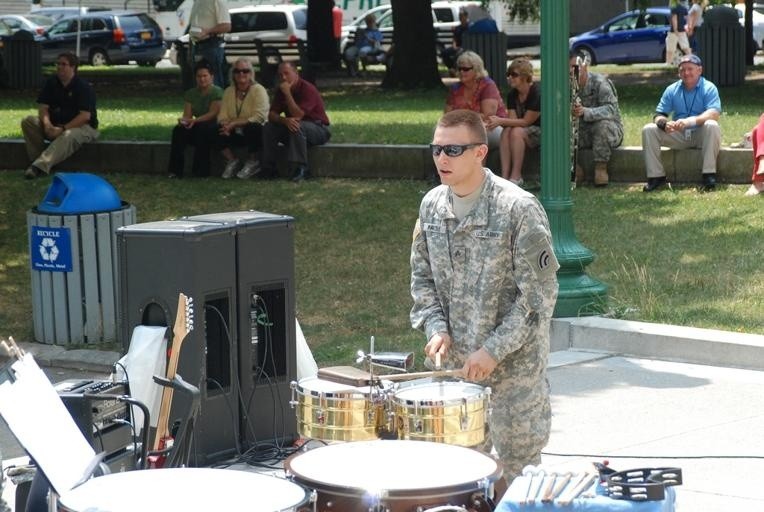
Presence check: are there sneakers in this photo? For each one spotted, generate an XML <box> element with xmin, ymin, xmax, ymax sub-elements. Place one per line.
<box><xmin>575</xmin><ymin>165</ymin><xmax>585</xmax><ymax>181</ymax></box>
<box><xmin>25</xmin><ymin>167</ymin><xmax>38</xmax><ymax>178</ymax></box>
<box><xmin>594</xmin><ymin>167</ymin><xmax>609</xmax><ymax>185</ymax></box>
<box><xmin>643</xmin><ymin>177</ymin><xmax>666</xmax><ymax>191</ymax></box>
<box><xmin>237</xmin><ymin>161</ymin><xmax>261</xmax><ymax>180</ymax></box>
<box><xmin>509</xmin><ymin>178</ymin><xmax>524</xmax><ymax>186</ymax></box>
<box><xmin>744</xmin><ymin>166</ymin><xmax>764</xmax><ymax>196</ymax></box>
<box><xmin>222</xmin><ymin>159</ymin><xmax>240</xmax><ymax>179</ymax></box>
<box><xmin>704</xmin><ymin>175</ymin><xmax>715</xmax><ymax>186</ymax></box>
<box><xmin>291</xmin><ymin>167</ymin><xmax>309</xmax><ymax>182</ymax></box>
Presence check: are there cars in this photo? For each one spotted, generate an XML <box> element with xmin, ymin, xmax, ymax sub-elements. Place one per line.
<box><xmin>685</xmin><ymin>4</ymin><xmax>764</xmax><ymax>54</ymax></box>
<box><xmin>569</xmin><ymin>7</ymin><xmax>690</xmax><ymax>66</ymax></box>
<box><xmin>348</xmin><ymin>4</ymin><xmax>392</xmax><ymax>26</ymax></box>
<box><xmin>0</xmin><ymin>15</ymin><xmax>56</xmax><ymax>37</ymax></box>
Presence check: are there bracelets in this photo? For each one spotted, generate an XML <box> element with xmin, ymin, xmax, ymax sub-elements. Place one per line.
<box><xmin>686</xmin><ymin>116</ymin><xmax>697</xmax><ymax>129</ymax></box>
<box><xmin>654</xmin><ymin>118</ymin><xmax>669</xmax><ymax>132</ymax></box>
<box><xmin>59</xmin><ymin>123</ymin><xmax>67</xmax><ymax>132</ymax></box>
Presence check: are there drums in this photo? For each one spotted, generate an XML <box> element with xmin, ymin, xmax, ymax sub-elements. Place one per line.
<box><xmin>290</xmin><ymin>374</ymin><xmax>388</xmax><ymax>441</ymax></box>
<box><xmin>390</xmin><ymin>382</ymin><xmax>487</xmax><ymax>448</ymax></box>
<box><xmin>288</xmin><ymin>440</ymin><xmax>505</xmax><ymax>511</ymax></box>
<box><xmin>58</xmin><ymin>468</ymin><xmax>309</xmax><ymax>512</ymax></box>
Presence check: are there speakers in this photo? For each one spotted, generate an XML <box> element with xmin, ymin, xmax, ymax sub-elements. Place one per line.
<box><xmin>116</xmin><ymin>220</ymin><xmax>240</xmax><ymax>468</ymax></box>
<box><xmin>181</xmin><ymin>209</ymin><xmax>299</xmax><ymax>456</ymax></box>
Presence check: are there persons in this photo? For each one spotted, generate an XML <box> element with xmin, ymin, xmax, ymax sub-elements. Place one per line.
<box><xmin>685</xmin><ymin>0</ymin><xmax>709</xmax><ymax>49</ymax></box>
<box><xmin>740</xmin><ymin>112</ymin><xmax>763</xmax><ymax>201</ymax></box>
<box><xmin>28</xmin><ymin>1</ymin><xmax>44</xmax><ymax>15</ymax></box>
<box><xmin>407</xmin><ymin>107</ymin><xmax>559</xmax><ymax>489</ymax></box>
<box><xmin>640</xmin><ymin>53</ymin><xmax>724</xmax><ymax>193</ymax></box>
<box><xmin>570</xmin><ymin>49</ymin><xmax>624</xmax><ymax>188</ymax></box>
<box><xmin>440</xmin><ymin>8</ymin><xmax>472</xmax><ymax>78</ymax></box>
<box><xmin>665</xmin><ymin>0</ymin><xmax>691</xmax><ymax>65</ymax></box>
<box><xmin>486</xmin><ymin>57</ymin><xmax>539</xmax><ymax>186</ymax></box>
<box><xmin>166</xmin><ymin>59</ymin><xmax>223</xmax><ymax>180</ymax></box>
<box><xmin>16</xmin><ymin>46</ymin><xmax>100</xmax><ymax>180</ymax></box>
<box><xmin>260</xmin><ymin>61</ymin><xmax>332</xmax><ymax>182</ymax></box>
<box><xmin>342</xmin><ymin>12</ymin><xmax>383</xmax><ymax>79</ymax></box>
<box><xmin>332</xmin><ymin>0</ymin><xmax>343</xmax><ymax>69</ymax></box>
<box><xmin>216</xmin><ymin>60</ymin><xmax>269</xmax><ymax>180</ymax></box>
<box><xmin>444</xmin><ymin>49</ymin><xmax>506</xmax><ymax>152</ymax></box>
<box><xmin>185</xmin><ymin>1</ymin><xmax>233</xmax><ymax>91</ymax></box>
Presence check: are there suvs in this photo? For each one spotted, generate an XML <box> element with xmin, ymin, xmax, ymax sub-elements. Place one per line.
<box><xmin>341</xmin><ymin>1</ymin><xmax>486</xmax><ymax>69</ymax></box>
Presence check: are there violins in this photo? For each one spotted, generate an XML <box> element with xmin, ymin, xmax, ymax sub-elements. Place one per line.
<box><xmin>145</xmin><ymin>293</ymin><xmax>194</xmax><ymax>469</ymax></box>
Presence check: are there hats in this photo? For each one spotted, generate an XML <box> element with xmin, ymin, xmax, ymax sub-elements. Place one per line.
<box><xmin>678</xmin><ymin>54</ymin><xmax>701</xmax><ymax>67</ymax></box>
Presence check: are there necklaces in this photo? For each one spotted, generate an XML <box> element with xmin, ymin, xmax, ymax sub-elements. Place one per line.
<box><xmin>237</xmin><ymin>86</ymin><xmax>249</xmax><ymax>97</ymax></box>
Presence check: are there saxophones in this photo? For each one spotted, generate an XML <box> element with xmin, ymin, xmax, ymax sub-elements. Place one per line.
<box><xmin>570</xmin><ymin>54</ymin><xmax>587</xmax><ymax>191</ymax></box>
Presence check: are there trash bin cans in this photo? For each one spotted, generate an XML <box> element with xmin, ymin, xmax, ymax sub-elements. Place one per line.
<box><xmin>3</xmin><ymin>30</ymin><xmax>41</xmax><ymax>89</ymax></box>
<box><xmin>695</xmin><ymin>7</ymin><xmax>745</xmax><ymax>87</ymax></box>
<box><xmin>27</xmin><ymin>172</ymin><xmax>137</xmax><ymax>346</ymax></box>
<box><xmin>463</xmin><ymin>18</ymin><xmax>507</xmax><ymax>91</ymax></box>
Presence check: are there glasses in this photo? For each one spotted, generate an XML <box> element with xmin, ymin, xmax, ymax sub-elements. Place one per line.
<box><xmin>506</xmin><ymin>71</ymin><xmax>519</xmax><ymax>77</ymax></box>
<box><xmin>458</xmin><ymin>66</ymin><xmax>471</xmax><ymax>71</ymax></box>
<box><xmin>233</xmin><ymin>67</ymin><xmax>252</xmax><ymax>75</ymax></box>
<box><xmin>56</xmin><ymin>60</ymin><xmax>67</xmax><ymax>67</ymax></box>
<box><xmin>428</xmin><ymin>142</ymin><xmax>486</xmax><ymax>157</ymax></box>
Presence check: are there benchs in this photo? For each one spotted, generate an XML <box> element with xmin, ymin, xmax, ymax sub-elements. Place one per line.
<box><xmin>346</xmin><ymin>29</ymin><xmax>454</xmax><ymax>71</ymax></box>
<box><xmin>177</xmin><ymin>38</ymin><xmax>330</xmax><ymax>82</ymax></box>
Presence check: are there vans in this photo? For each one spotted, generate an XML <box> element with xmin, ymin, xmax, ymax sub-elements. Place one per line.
<box><xmin>36</xmin><ymin>13</ymin><xmax>166</xmax><ymax>67</ymax></box>
<box><xmin>26</xmin><ymin>7</ymin><xmax>111</xmax><ymax>17</ymax></box>
<box><xmin>169</xmin><ymin>5</ymin><xmax>307</xmax><ymax>68</ymax></box>
